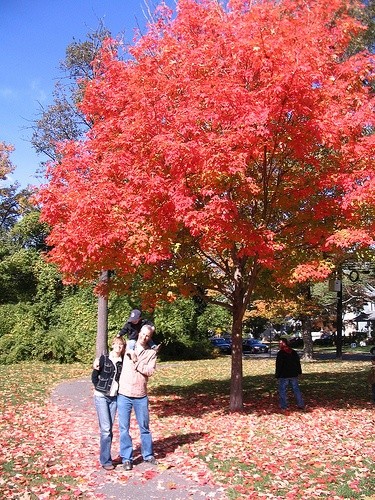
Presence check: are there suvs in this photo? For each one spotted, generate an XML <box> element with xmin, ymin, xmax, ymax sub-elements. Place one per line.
<box><xmin>207</xmin><ymin>338</ymin><xmax>231</xmax><ymax>354</ymax></box>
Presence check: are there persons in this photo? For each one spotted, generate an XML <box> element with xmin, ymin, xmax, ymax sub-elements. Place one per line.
<box><xmin>93</xmin><ymin>324</ymin><xmax>160</xmax><ymax>470</ymax></box>
<box><xmin>91</xmin><ymin>337</ymin><xmax>126</xmax><ymax>470</ymax></box>
<box><xmin>274</xmin><ymin>337</ymin><xmax>307</xmax><ymax>414</ymax></box>
<box><xmin>118</xmin><ymin>309</ymin><xmax>163</xmax><ymax>360</ymax></box>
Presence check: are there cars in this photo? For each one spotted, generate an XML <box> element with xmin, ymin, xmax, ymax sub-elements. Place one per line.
<box><xmin>242</xmin><ymin>338</ymin><xmax>269</xmax><ymax>354</ymax></box>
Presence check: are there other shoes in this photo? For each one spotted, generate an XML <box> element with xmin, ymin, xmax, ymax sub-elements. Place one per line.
<box><xmin>155</xmin><ymin>344</ymin><xmax>161</xmax><ymax>352</ymax></box>
<box><xmin>147</xmin><ymin>457</ymin><xmax>158</xmax><ymax>465</ymax></box>
<box><xmin>123</xmin><ymin>462</ymin><xmax>132</xmax><ymax>470</ymax></box>
<box><xmin>102</xmin><ymin>464</ymin><xmax>114</xmax><ymax>470</ymax></box>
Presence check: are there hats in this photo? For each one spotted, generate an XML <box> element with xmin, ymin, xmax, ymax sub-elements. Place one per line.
<box><xmin>128</xmin><ymin>309</ymin><xmax>142</xmax><ymax>322</ymax></box>
